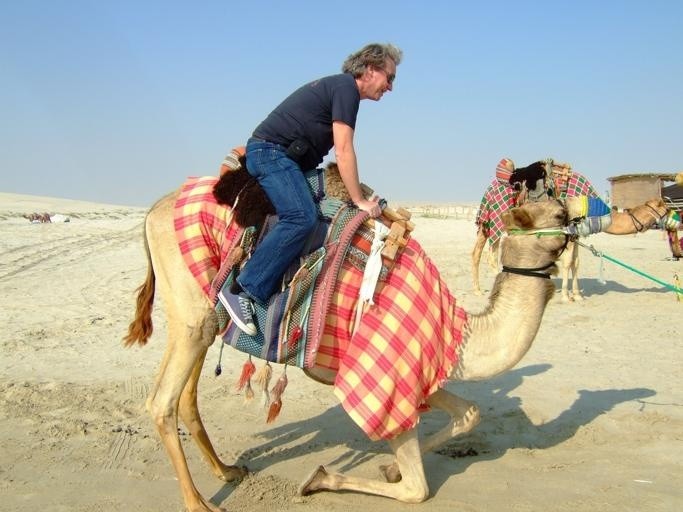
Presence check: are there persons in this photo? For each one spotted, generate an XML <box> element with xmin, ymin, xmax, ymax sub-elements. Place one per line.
<box><xmin>217</xmin><ymin>42</ymin><xmax>402</xmax><ymax>337</ymax></box>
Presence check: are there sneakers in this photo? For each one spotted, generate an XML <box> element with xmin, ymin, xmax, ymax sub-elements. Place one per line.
<box><xmin>217</xmin><ymin>286</ymin><xmax>259</xmax><ymax>336</ymax></box>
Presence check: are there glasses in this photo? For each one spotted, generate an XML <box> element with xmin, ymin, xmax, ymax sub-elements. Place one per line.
<box><xmin>370</xmin><ymin>64</ymin><xmax>395</xmax><ymax>84</ymax></box>
